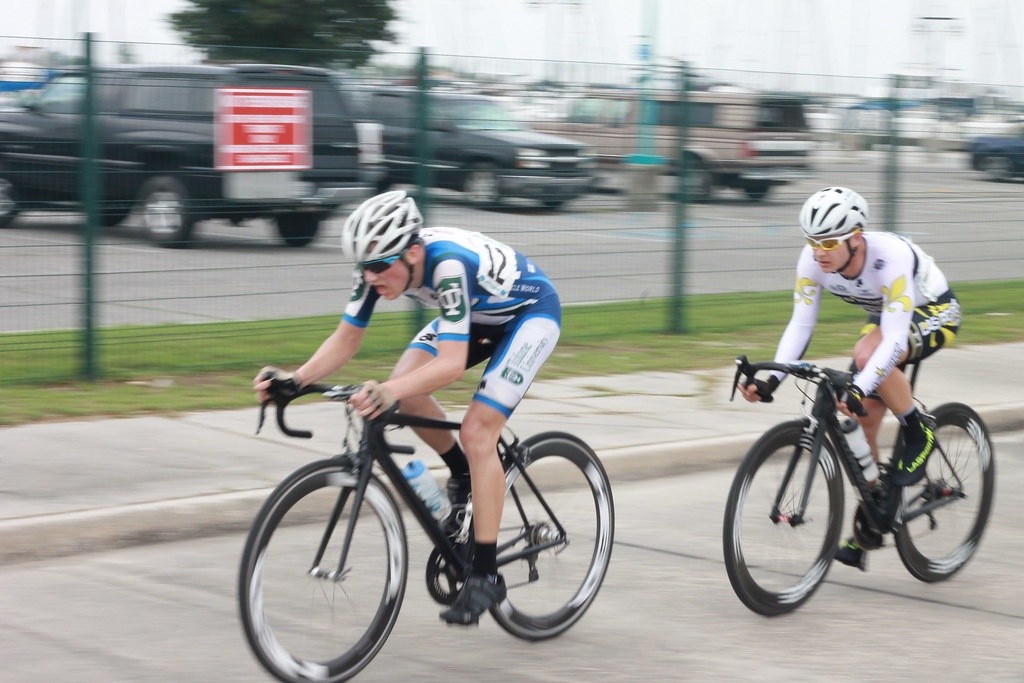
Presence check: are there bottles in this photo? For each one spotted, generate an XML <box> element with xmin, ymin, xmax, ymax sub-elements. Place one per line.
<box><xmin>402</xmin><ymin>459</ymin><xmax>454</xmax><ymax>522</ymax></box>
<box><xmin>841</xmin><ymin>418</ymin><xmax>880</xmax><ymax>482</ymax></box>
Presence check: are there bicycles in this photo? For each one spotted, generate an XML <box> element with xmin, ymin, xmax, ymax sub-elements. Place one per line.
<box><xmin>720</xmin><ymin>342</ymin><xmax>997</xmax><ymax>618</ymax></box>
<box><xmin>236</xmin><ymin>368</ymin><xmax>615</xmax><ymax>683</ymax></box>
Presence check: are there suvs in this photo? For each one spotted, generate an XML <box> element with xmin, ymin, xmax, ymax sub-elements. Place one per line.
<box><xmin>525</xmin><ymin>83</ymin><xmax>811</xmax><ymax>202</ymax></box>
<box><xmin>347</xmin><ymin>84</ymin><xmax>600</xmax><ymax>215</ymax></box>
<box><xmin>0</xmin><ymin>58</ymin><xmax>382</xmax><ymax>250</ymax></box>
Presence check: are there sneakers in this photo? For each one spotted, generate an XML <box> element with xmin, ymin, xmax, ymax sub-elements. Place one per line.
<box><xmin>834</xmin><ymin>536</ymin><xmax>865</xmax><ymax>570</ymax></box>
<box><xmin>441</xmin><ymin>475</ymin><xmax>472</xmax><ymax>538</ymax></box>
<box><xmin>440</xmin><ymin>574</ymin><xmax>508</xmax><ymax>623</ymax></box>
<box><xmin>894</xmin><ymin>416</ymin><xmax>938</xmax><ymax>487</ymax></box>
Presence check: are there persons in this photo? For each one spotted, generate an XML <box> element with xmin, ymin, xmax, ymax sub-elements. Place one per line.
<box><xmin>733</xmin><ymin>185</ymin><xmax>964</xmax><ymax>571</ymax></box>
<box><xmin>252</xmin><ymin>189</ymin><xmax>562</xmax><ymax>625</ymax></box>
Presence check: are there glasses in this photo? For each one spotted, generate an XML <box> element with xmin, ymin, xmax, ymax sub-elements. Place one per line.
<box><xmin>804</xmin><ymin>228</ymin><xmax>861</xmax><ymax>250</ymax></box>
<box><xmin>356</xmin><ymin>238</ymin><xmax>421</xmax><ymax>274</ymax></box>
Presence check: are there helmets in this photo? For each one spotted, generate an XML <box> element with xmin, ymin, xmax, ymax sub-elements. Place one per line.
<box><xmin>342</xmin><ymin>190</ymin><xmax>424</xmax><ymax>262</ymax></box>
<box><xmin>799</xmin><ymin>186</ymin><xmax>869</xmax><ymax>237</ymax></box>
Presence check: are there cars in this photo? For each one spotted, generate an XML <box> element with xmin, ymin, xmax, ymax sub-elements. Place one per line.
<box><xmin>971</xmin><ymin>116</ymin><xmax>1024</xmax><ymax>185</ymax></box>
